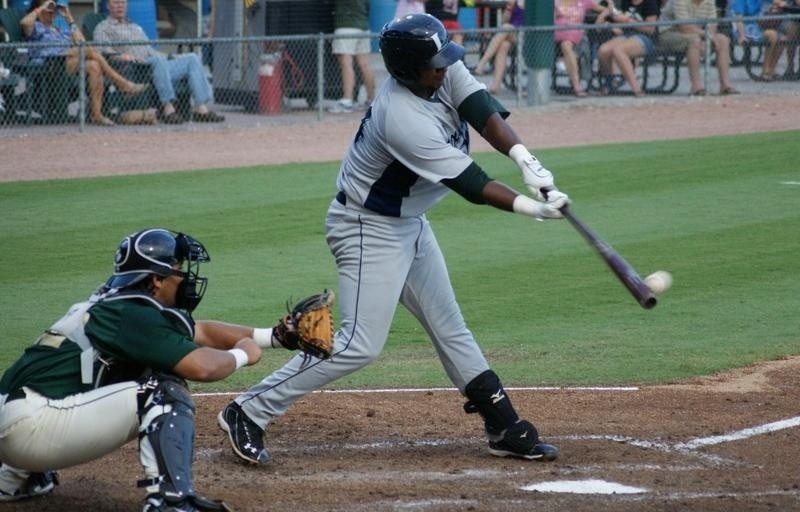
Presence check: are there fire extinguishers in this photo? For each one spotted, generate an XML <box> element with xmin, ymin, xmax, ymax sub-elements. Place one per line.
<box><xmin>258</xmin><ymin>39</ymin><xmax>304</xmax><ymax>116</ymax></box>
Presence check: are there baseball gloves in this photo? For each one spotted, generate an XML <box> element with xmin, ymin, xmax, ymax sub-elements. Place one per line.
<box><xmin>273</xmin><ymin>289</ymin><xmax>335</xmax><ymax>360</ymax></box>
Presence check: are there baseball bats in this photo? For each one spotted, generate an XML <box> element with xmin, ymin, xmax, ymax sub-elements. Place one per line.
<box><xmin>540</xmin><ymin>185</ymin><xmax>657</xmax><ymax>309</ymax></box>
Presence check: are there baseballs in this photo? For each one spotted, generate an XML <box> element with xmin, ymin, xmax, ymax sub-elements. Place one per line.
<box><xmin>644</xmin><ymin>271</ymin><xmax>673</xmax><ymax>294</ymax></box>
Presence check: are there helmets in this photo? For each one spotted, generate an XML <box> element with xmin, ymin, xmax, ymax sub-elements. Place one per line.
<box><xmin>379</xmin><ymin>13</ymin><xmax>464</xmax><ymax>69</ymax></box>
<box><xmin>105</xmin><ymin>228</ymin><xmax>210</xmax><ymax>310</ymax></box>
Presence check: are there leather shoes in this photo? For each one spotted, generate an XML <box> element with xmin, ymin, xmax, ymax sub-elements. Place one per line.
<box><xmin>165</xmin><ymin>111</ymin><xmax>224</xmax><ymax>123</ymax></box>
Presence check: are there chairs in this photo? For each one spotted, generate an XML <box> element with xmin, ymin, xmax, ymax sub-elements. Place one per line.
<box><xmin>0</xmin><ymin>1</ymin><xmax>212</xmax><ymax>127</ymax></box>
<box><xmin>438</xmin><ymin>0</ymin><xmax>800</xmax><ymax>97</ymax></box>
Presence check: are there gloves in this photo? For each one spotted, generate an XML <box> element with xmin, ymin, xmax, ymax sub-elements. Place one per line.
<box><xmin>509</xmin><ymin>144</ymin><xmax>572</xmax><ymax>219</ymax></box>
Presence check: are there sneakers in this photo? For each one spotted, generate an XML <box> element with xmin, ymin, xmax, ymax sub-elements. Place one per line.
<box><xmin>217</xmin><ymin>401</ymin><xmax>270</xmax><ymax>464</ymax></box>
<box><xmin>328</xmin><ymin>102</ymin><xmax>352</xmax><ymax>113</ymax></box>
<box><xmin>488</xmin><ymin>420</ymin><xmax>557</xmax><ymax>461</ymax></box>
<box><xmin>0</xmin><ymin>473</ymin><xmax>53</xmax><ymax>501</ymax></box>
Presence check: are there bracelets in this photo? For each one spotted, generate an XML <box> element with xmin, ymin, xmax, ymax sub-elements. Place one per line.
<box><xmin>227</xmin><ymin>346</ymin><xmax>250</xmax><ymax>371</ymax></box>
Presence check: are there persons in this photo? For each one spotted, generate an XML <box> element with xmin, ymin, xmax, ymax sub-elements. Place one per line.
<box><xmin>1</xmin><ymin>1</ymin><xmax>226</xmax><ymax>127</ymax></box>
<box><xmin>214</xmin><ymin>9</ymin><xmax>574</xmax><ymax>472</ymax></box>
<box><xmin>327</xmin><ymin>1</ymin><xmax>800</xmax><ymax>114</ymax></box>
<box><xmin>1</xmin><ymin>225</ymin><xmax>338</xmax><ymax>509</ymax></box>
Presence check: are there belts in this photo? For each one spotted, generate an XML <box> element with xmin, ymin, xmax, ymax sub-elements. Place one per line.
<box><xmin>4</xmin><ymin>391</ymin><xmax>26</xmax><ymax>405</ymax></box>
